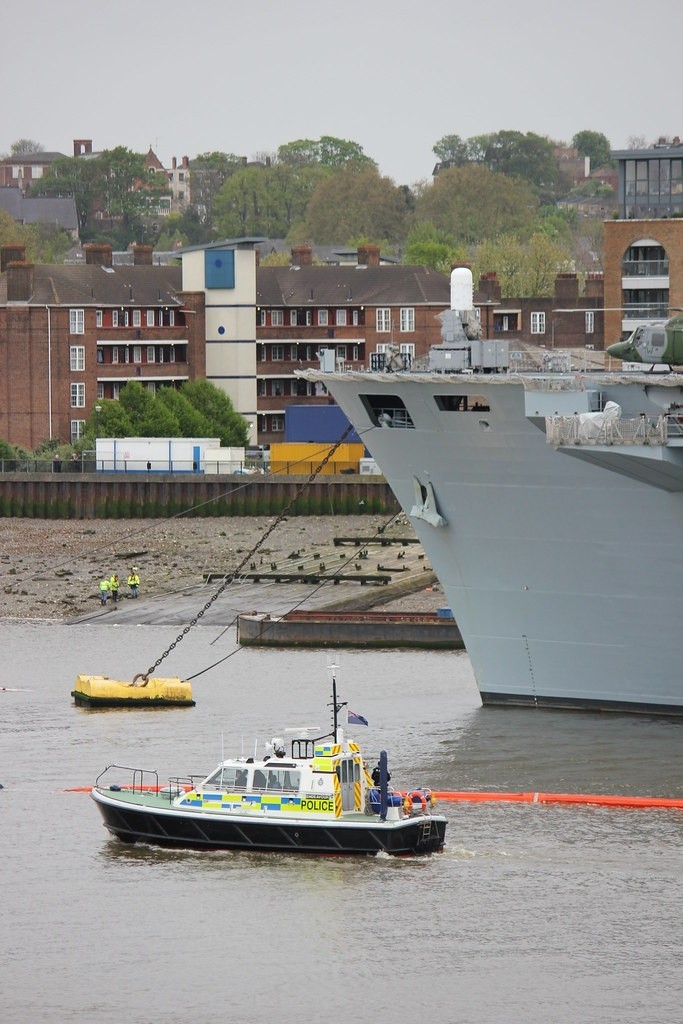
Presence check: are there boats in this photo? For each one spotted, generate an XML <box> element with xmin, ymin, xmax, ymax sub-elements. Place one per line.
<box><xmin>238</xmin><ymin>610</ymin><xmax>466</xmax><ymax>649</ymax></box>
<box><xmin>88</xmin><ymin>677</ymin><xmax>450</xmax><ymax>861</ymax></box>
<box><xmin>293</xmin><ymin>267</ymin><xmax>683</xmax><ymax>717</ymax></box>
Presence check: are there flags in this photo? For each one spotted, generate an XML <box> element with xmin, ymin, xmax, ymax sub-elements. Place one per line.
<box><xmin>348</xmin><ymin>711</ymin><xmax>369</xmax><ymax>726</ymax></box>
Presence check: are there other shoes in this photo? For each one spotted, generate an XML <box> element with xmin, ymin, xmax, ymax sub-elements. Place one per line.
<box><xmin>112</xmin><ymin>598</ymin><xmax>118</xmax><ymax>602</ymax></box>
<box><xmin>101</xmin><ymin>601</ymin><xmax>107</xmax><ymax>606</ymax></box>
<box><xmin>131</xmin><ymin>595</ymin><xmax>139</xmax><ymax>599</ymax></box>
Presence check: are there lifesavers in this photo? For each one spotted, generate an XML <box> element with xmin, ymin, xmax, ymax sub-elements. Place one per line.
<box><xmin>405</xmin><ymin>792</ymin><xmax>427</xmax><ymax>814</ymax></box>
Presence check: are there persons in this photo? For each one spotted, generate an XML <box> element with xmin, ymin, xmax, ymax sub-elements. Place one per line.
<box><xmin>99</xmin><ymin>575</ymin><xmax>110</xmax><ymax>606</ymax></box>
<box><xmin>128</xmin><ymin>572</ymin><xmax>140</xmax><ymax>598</ymax></box>
<box><xmin>235</xmin><ymin>770</ymin><xmax>280</xmax><ymax>792</ymax></box>
<box><xmin>110</xmin><ymin>574</ymin><xmax>119</xmax><ymax>602</ymax></box>
